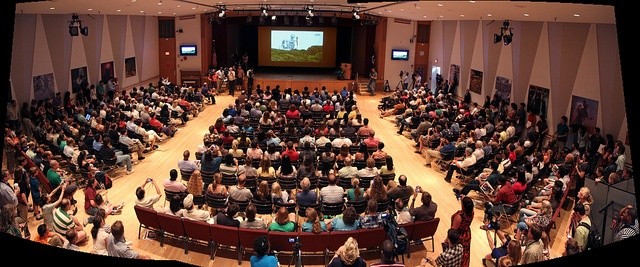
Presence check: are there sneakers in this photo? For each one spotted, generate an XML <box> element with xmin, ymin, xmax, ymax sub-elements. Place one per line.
<box><xmin>118</xmin><ymin>165</ymin><xmax>127</xmax><ymax>170</ymax></box>
<box><xmin>126</xmin><ymin>169</ymin><xmax>133</xmax><ymax>174</ymax></box>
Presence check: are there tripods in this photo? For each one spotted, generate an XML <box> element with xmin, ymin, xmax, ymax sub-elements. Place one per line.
<box><xmin>491</xmin><ymin>228</ymin><xmax>512</xmax><ymax>247</ymax></box>
<box><xmin>392</xmin><ymin>253</ymin><xmax>404</xmax><ymax>265</ymax></box>
<box><xmin>289</xmin><ymin>251</ymin><xmax>304</xmax><ymax>267</ymax></box>
<box><xmin>408</xmin><ymin>81</ymin><xmax>417</xmax><ymax>90</ymax></box>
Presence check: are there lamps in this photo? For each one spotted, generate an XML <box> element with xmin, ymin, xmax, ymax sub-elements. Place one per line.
<box><xmin>494</xmin><ymin>20</ymin><xmax>513</xmax><ymax>45</ymax></box>
<box><xmin>308</xmin><ymin>9</ymin><xmax>314</xmax><ymax>17</ymax></box>
<box><xmin>262</xmin><ymin>9</ymin><xmax>269</xmax><ymax>17</ymax></box>
<box><xmin>218</xmin><ymin>10</ymin><xmax>225</xmax><ymax>18</ymax></box>
<box><xmin>353</xmin><ymin>11</ymin><xmax>361</xmax><ymax>20</ymax></box>
<box><xmin>68</xmin><ymin>14</ymin><xmax>89</xmax><ymax>37</ymax></box>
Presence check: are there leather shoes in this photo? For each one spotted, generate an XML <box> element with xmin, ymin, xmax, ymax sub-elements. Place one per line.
<box><xmin>143</xmin><ymin>149</ymin><xmax>151</xmax><ymax>154</ymax></box>
<box><xmin>444</xmin><ymin>178</ymin><xmax>450</xmax><ymax>183</ymax></box>
<box><xmin>397</xmin><ymin>131</ymin><xmax>402</xmax><ymax>134</ymax></box>
<box><xmin>424</xmin><ymin>164</ymin><xmax>431</xmax><ymax>167</ymax></box>
<box><xmin>228</xmin><ymin>93</ymin><xmax>231</xmax><ymax>95</ymax></box>
<box><xmin>138</xmin><ymin>157</ymin><xmax>145</xmax><ymax>160</ymax></box>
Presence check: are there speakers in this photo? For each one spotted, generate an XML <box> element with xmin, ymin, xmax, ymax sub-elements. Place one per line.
<box><xmin>493</xmin><ymin>32</ymin><xmax>502</xmax><ymax>45</ymax></box>
<box><xmin>69</xmin><ymin>26</ymin><xmax>79</xmax><ymax>37</ymax></box>
<box><xmin>503</xmin><ymin>34</ymin><xmax>512</xmax><ymax>46</ymax></box>
<box><xmin>80</xmin><ymin>26</ymin><xmax>88</xmax><ymax>36</ymax></box>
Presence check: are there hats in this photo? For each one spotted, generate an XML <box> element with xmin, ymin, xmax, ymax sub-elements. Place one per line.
<box><xmin>30</xmin><ymin>167</ymin><xmax>36</xmax><ymax>173</ymax></box>
<box><xmin>183</xmin><ymin>193</ymin><xmax>194</xmax><ymax>209</ymax></box>
<box><xmin>519</xmin><ymin>222</ymin><xmax>528</xmax><ymax>231</ymax></box>
<box><xmin>300</xmin><ymin>177</ymin><xmax>310</xmax><ymax>189</ymax></box>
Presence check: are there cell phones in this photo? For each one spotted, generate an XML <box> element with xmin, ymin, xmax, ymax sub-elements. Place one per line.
<box><xmin>147</xmin><ymin>179</ymin><xmax>152</xmax><ymax>181</ymax></box>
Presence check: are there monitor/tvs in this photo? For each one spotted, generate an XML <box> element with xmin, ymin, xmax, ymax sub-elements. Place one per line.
<box><xmin>391</xmin><ymin>49</ymin><xmax>409</xmax><ymax>61</ymax></box>
<box><xmin>179</xmin><ymin>45</ymin><xmax>197</xmax><ymax>56</ymax></box>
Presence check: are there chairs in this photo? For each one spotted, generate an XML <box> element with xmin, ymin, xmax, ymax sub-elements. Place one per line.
<box><xmin>163</xmin><ymin>104</ymin><xmax>411</xmax><ymax>216</ymax></box>
<box><xmin>467</xmin><ymin>127</ymin><xmax>583</xmax><ymax>243</ymax></box>
<box><xmin>329</xmin><ymin>230</ymin><xmax>357</xmax><ymax>257</ymax></box>
<box><xmin>239</xmin><ymin>227</ymin><xmax>267</xmax><ymax>266</ymax></box>
<box><xmin>386</xmin><ymin>222</ymin><xmax>413</xmax><ymax>241</ymax></box>
<box><xmin>134</xmin><ymin>204</ymin><xmax>162</xmax><ymax>247</ymax></box>
<box><xmin>357</xmin><ymin>226</ymin><xmax>387</xmax><ymax>258</ymax></box>
<box><xmin>268</xmin><ymin>231</ymin><xmax>300</xmax><ymax>255</ymax></box>
<box><xmin>300</xmin><ymin>231</ymin><xmax>328</xmax><ymax>265</ymax></box>
<box><xmin>156</xmin><ymin>212</ymin><xmax>186</xmax><ymax>254</ymax></box>
<box><xmin>182</xmin><ymin>216</ymin><xmax>212</xmax><ymax>260</ymax></box>
<box><xmin>16</xmin><ymin>146</ymin><xmax>55</xmax><ymax>200</ymax></box>
<box><xmin>414</xmin><ymin>217</ymin><xmax>440</xmax><ymax>252</ymax></box>
<box><xmin>212</xmin><ymin>223</ymin><xmax>240</xmax><ymax>265</ymax></box>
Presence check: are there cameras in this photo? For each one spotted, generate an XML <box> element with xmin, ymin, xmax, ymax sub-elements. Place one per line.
<box><xmin>486</xmin><ymin>218</ymin><xmax>501</xmax><ymax>231</ymax></box>
<box><xmin>381</xmin><ymin>213</ymin><xmax>408</xmax><ymax>255</ymax></box>
<box><xmin>288</xmin><ymin>235</ymin><xmax>303</xmax><ymax>249</ymax></box>
<box><xmin>224</xmin><ymin>67</ymin><xmax>229</xmax><ymax>77</ymax></box>
<box><xmin>411</xmin><ymin>74</ymin><xmax>417</xmax><ymax>81</ymax></box>
<box><xmin>436</xmin><ymin>74</ymin><xmax>443</xmax><ymax>83</ymax></box>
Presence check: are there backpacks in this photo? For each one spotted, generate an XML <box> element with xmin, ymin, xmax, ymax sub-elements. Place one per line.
<box><xmin>577</xmin><ymin>215</ymin><xmax>601</xmax><ymax>251</ymax></box>
